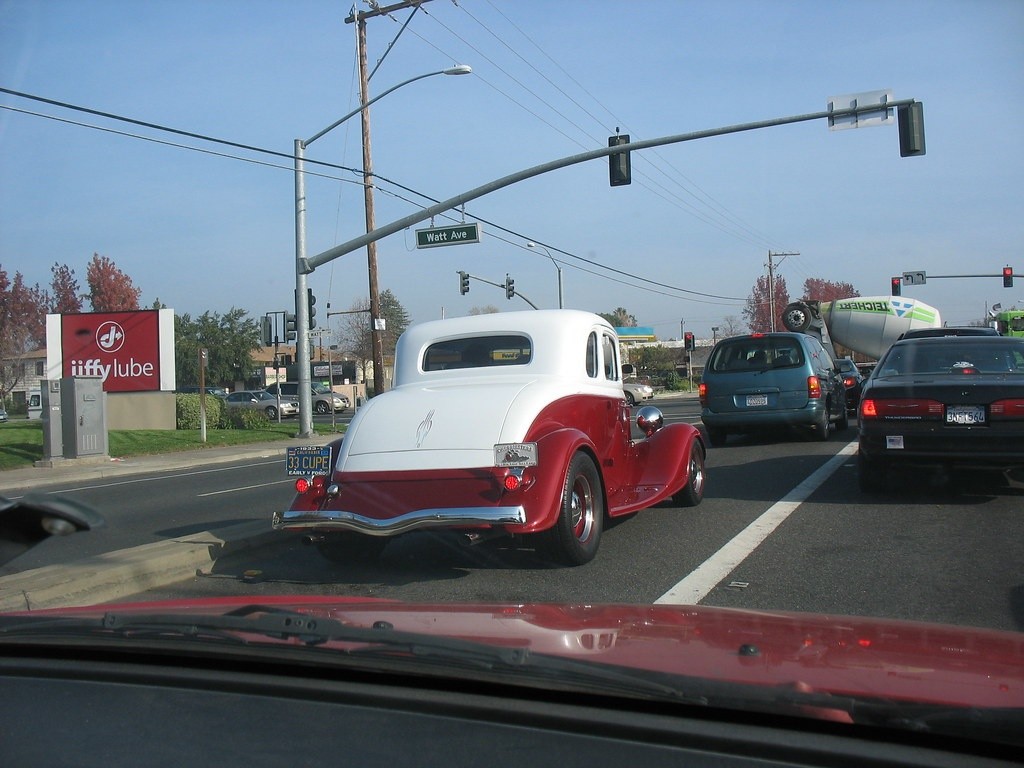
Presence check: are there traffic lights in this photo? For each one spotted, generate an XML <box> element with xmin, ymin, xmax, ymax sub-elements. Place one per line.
<box><xmin>278</xmin><ymin>310</ymin><xmax>297</xmax><ymax>343</ymax></box>
<box><xmin>891</xmin><ymin>277</ymin><xmax>901</xmax><ymax>296</ymax></box>
<box><xmin>294</xmin><ymin>288</ymin><xmax>317</xmax><ymax>330</ymax></box>
<box><xmin>684</xmin><ymin>356</ymin><xmax>690</xmax><ymax>362</ymax></box>
<box><xmin>1003</xmin><ymin>266</ymin><xmax>1014</xmax><ymax>287</ymax></box>
<box><xmin>506</xmin><ymin>276</ymin><xmax>515</xmax><ymax>299</ymax></box>
<box><xmin>684</xmin><ymin>331</ymin><xmax>693</xmax><ymax>350</ymax></box>
<box><xmin>459</xmin><ymin>269</ymin><xmax>470</xmax><ymax>296</ymax></box>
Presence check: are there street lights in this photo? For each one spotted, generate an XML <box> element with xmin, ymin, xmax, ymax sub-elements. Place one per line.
<box><xmin>525</xmin><ymin>242</ymin><xmax>564</xmax><ymax>312</ymax></box>
<box><xmin>294</xmin><ymin>62</ymin><xmax>474</xmax><ymax>436</ymax></box>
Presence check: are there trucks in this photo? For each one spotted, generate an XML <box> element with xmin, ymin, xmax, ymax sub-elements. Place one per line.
<box><xmin>987</xmin><ymin>310</ymin><xmax>1024</xmax><ymax>369</ymax></box>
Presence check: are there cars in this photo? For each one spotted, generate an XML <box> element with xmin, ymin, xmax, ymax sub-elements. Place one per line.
<box><xmin>830</xmin><ymin>359</ymin><xmax>884</xmax><ymax>414</ymax></box>
<box><xmin>623</xmin><ymin>383</ymin><xmax>655</xmax><ymax>406</ymax></box>
<box><xmin>221</xmin><ymin>390</ymin><xmax>301</xmax><ymax>420</ymax></box>
<box><xmin>858</xmin><ymin>335</ymin><xmax>1024</xmax><ymax>490</ymax></box>
<box><xmin>264</xmin><ymin>382</ymin><xmax>351</xmax><ymax>414</ymax></box>
<box><xmin>274</xmin><ymin>306</ymin><xmax>707</xmax><ymax>563</ymax></box>
<box><xmin>897</xmin><ymin>325</ymin><xmax>1015</xmax><ymax>374</ymax></box>
<box><xmin>698</xmin><ymin>331</ymin><xmax>852</xmax><ymax>441</ymax></box>
<box><xmin>172</xmin><ymin>385</ymin><xmax>228</xmax><ymax>401</ymax></box>
<box><xmin>0</xmin><ymin>409</ymin><xmax>8</xmax><ymax>422</ymax></box>
